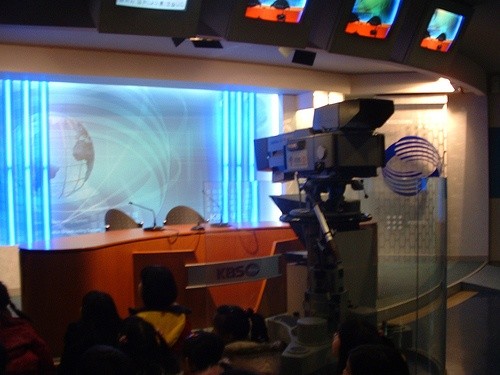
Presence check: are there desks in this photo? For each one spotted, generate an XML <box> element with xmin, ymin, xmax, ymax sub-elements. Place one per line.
<box><xmin>19</xmin><ymin>219</ymin><xmax>305</xmax><ymax>357</ymax></box>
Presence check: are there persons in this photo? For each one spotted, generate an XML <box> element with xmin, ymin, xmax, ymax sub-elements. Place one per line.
<box><xmin>60</xmin><ymin>265</ymin><xmax>404</xmax><ymax>375</ymax></box>
<box><xmin>0</xmin><ymin>278</ymin><xmax>54</xmax><ymax>375</ymax></box>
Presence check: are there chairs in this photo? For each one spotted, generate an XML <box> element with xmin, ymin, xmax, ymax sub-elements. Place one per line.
<box><xmin>104</xmin><ymin>208</ymin><xmax>141</xmax><ymax>233</ymax></box>
<box><xmin>165</xmin><ymin>205</ymin><xmax>206</xmax><ymax>226</ymax></box>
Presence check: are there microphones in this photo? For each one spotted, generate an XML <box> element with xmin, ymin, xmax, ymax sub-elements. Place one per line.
<box><xmin>202</xmin><ymin>190</ymin><xmax>229</xmax><ymax>227</ymax></box>
<box><xmin>129</xmin><ymin>202</ymin><xmax>164</xmax><ymax>230</ymax></box>
